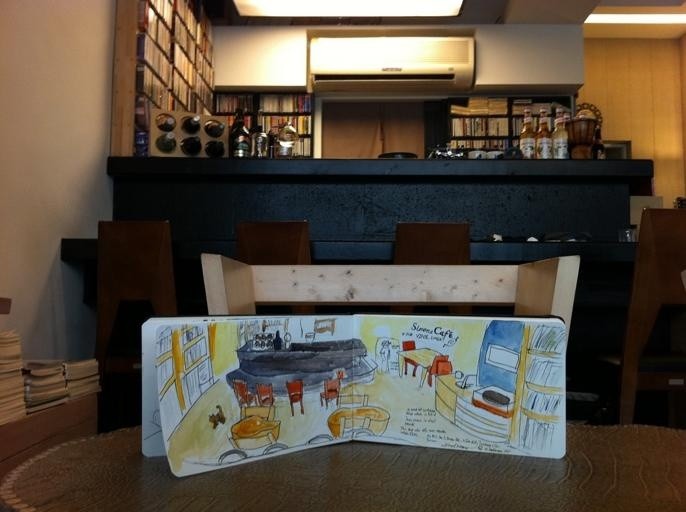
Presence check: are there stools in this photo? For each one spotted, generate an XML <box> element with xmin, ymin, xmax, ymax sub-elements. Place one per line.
<box><xmin>628</xmin><ymin>195</ymin><xmax>660</xmax><ymax>240</ymax></box>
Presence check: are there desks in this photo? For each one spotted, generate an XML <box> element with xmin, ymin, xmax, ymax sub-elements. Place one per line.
<box><xmin>3</xmin><ymin>420</ymin><xmax>686</xmax><ymax>510</ymax></box>
<box><xmin>326</xmin><ymin>406</ymin><xmax>389</xmax><ymax>438</ymax></box>
<box><xmin>229</xmin><ymin>415</ymin><xmax>281</xmax><ymax>441</ymax></box>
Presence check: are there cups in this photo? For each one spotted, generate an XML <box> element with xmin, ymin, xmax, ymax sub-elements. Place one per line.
<box><xmin>468</xmin><ymin>148</ymin><xmax>520</xmax><ymax>160</ymax></box>
<box><xmin>619</xmin><ymin>224</ymin><xmax>637</xmax><ymax>243</ymax></box>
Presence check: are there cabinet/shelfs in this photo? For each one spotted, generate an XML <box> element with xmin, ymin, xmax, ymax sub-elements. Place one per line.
<box><xmin>509</xmin><ymin>321</ymin><xmax>565</xmax><ymax>455</ymax></box>
<box><xmin>446</xmin><ymin>94</ymin><xmax>575</xmax><ymax>157</ymax></box>
<box><xmin>109</xmin><ymin>0</ymin><xmax>315</xmax><ymax>165</ymax></box>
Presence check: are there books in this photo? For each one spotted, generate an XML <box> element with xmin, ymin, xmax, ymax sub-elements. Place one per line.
<box><xmin>449</xmin><ymin>96</ymin><xmax>572</xmax><ymax>151</ymax></box>
<box><xmin>0</xmin><ymin>328</ymin><xmax>103</xmax><ymax>427</ymax></box>
<box><xmin>134</xmin><ymin>0</ymin><xmax>313</xmax><ymax>158</ymax></box>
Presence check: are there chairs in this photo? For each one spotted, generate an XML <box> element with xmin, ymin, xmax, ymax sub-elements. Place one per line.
<box><xmin>203</xmin><ymin>254</ymin><xmax>580</xmax><ymax>359</ymax></box>
<box><xmin>229</xmin><ymin>432</ymin><xmax>278</xmax><ymax>455</ymax></box>
<box><xmin>97</xmin><ymin>219</ymin><xmax>176</xmax><ymax>370</ymax></box>
<box><xmin>386</xmin><ymin>222</ymin><xmax>475</xmax><ymax>315</ymax></box>
<box><xmin>594</xmin><ymin>208</ymin><xmax>684</xmax><ymax>424</ymax></box>
<box><xmin>339</xmin><ymin>416</ymin><xmax>369</xmax><ymax>437</ymax></box>
<box><xmin>231</xmin><ymin>224</ymin><xmax>315</xmax><ymax>317</ymax></box>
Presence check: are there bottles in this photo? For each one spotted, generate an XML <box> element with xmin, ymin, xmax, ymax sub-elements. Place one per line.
<box><xmin>155</xmin><ymin>107</ymin><xmax>297</xmax><ymax>159</ymax></box>
<box><xmin>521</xmin><ymin>106</ymin><xmax>570</xmax><ymax>159</ymax></box>
<box><xmin>590</xmin><ymin>125</ymin><xmax>606</xmax><ymax>160</ymax></box>
<box><xmin>434</xmin><ymin>142</ymin><xmax>464</xmax><ymax>159</ymax></box>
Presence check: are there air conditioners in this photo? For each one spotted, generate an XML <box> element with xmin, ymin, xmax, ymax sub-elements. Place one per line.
<box><xmin>309</xmin><ymin>37</ymin><xmax>475</xmax><ymax>94</ymax></box>
